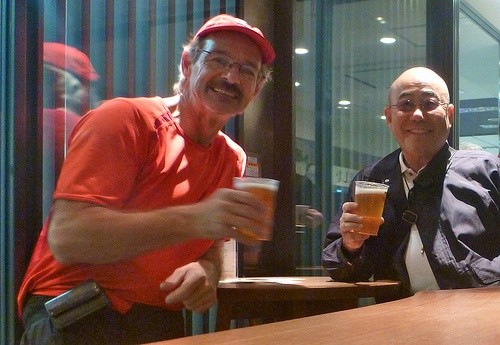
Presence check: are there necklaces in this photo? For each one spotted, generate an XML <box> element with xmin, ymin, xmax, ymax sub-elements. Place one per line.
<box><xmin>403</xmin><ymin>173</ymin><xmax>440</xmax><ymax>253</ymax></box>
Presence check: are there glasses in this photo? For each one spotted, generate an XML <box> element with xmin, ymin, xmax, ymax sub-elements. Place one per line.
<box><xmin>196</xmin><ymin>48</ymin><xmax>267</xmax><ymax>85</ymax></box>
<box><xmin>388</xmin><ymin>99</ymin><xmax>446</xmax><ymax>111</ymax></box>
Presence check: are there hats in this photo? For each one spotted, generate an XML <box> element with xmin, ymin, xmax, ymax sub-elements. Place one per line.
<box><xmin>192</xmin><ymin>13</ymin><xmax>276</xmax><ymax>66</ymax></box>
<box><xmin>42</xmin><ymin>39</ymin><xmax>99</xmax><ymax>84</ymax></box>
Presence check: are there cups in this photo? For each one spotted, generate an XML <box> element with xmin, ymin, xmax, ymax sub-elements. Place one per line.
<box><xmin>232</xmin><ymin>175</ymin><xmax>279</xmax><ymax>241</ymax></box>
<box><xmin>294</xmin><ymin>204</ymin><xmax>310</xmax><ymax>234</ymax></box>
<box><xmin>352</xmin><ymin>180</ymin><xmax>390</xmax><ymax>235</ymax></box>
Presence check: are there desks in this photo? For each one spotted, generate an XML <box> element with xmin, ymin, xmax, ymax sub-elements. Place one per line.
<box><xmin>209</xmin><ymin>277</ymin><xmax>400</xmax><ymax>332</ymax></box>
<box><xmin>135</xmin><ymin>287</ymin><xmax>500</xmax><ymax>345</ymax></box>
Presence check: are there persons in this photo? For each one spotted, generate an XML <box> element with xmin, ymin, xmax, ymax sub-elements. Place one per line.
<box><xmin>17</xmin><ymin>14</ymin><xmax>275</xmax><ymax>345</ymax></box>
<box><xmin>321</xmin><ymin>67</ymin><xmax>500</xmax><ymax>304</ymax></box>
<box><xmin>293</xmin><ymin>173</ymin><xmax>323</xmax><ymax>233</ymax></box>
<box><xmin>41</xmin><ymin>42</ymin><xmax>100</xmax><ymax>224</ymax></box>
